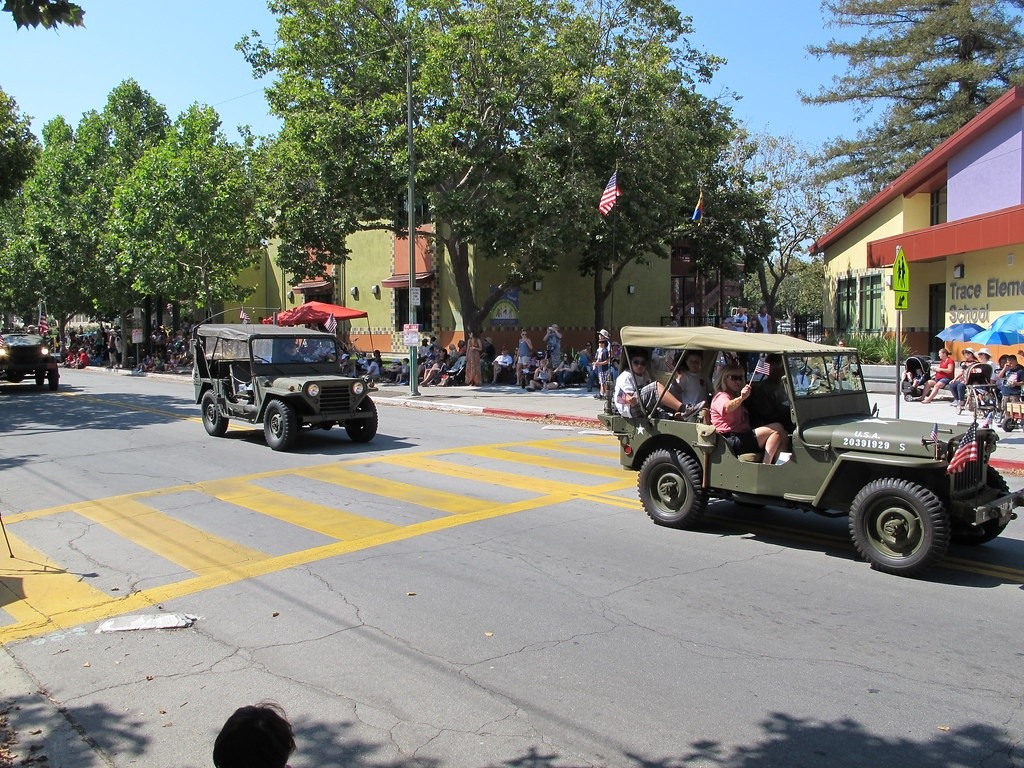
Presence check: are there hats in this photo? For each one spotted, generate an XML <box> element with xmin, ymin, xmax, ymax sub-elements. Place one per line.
<box><xmin>431</xmin><ymin>336</ymin><xmax>437</xmax><ymax>342</ymax></box>
<box><xmin>342</xmin><ymin>354</ymin><xmax>349</xmax><ymax>360</ymax></box>
<box><xmin>28</xmin><ymin>325</ymin><xmax>35</xmax><ymax>328</ymax></box>
<box><xmin>597</xmin><ymin>329</ymin><xmax>610</xmax><ymax>340</ymax></box>
<box><xmin>392</xmin><ymin>357</ymin><xmax>400</xmax><ymax>363</ymax></box>
<box><xmin>611</xmin><ymin>341</ymin><xmax>621</xmax><ymax>348</ymax></box>
<box><xmin>78</xmin><ymin>348</ymin><xmax>84</xmax><ymax>351</ymax></box>
<box><xmin>974</xmin><ymin>348</ymin><xmax>993</xmax><ymax>359</ymax></box>
<box><xmin>484</xmin><ymin>337</ymin><xmax>493</xmax><ymax>344</ymax></box>
<box><xmin>403</xmin><ymin>358</ymin><xmax>409</xmax><ymax>363</ymax></box>
<box><xmin>961</xmin><ymin>347</ymin><xmax>975</xmax><ymax>355</ymax></box>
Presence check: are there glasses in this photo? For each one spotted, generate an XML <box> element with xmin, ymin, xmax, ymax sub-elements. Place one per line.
<box><xmin>563</xmin><ymin>356</ymin><xmax>567</xmax><ymax>358</ymax></box>
<box><xmin>841</xmin><ymin>344</ymin><xmax>845</xmax><ymax>345</ymax></box>
<box><xmin>965</xmin><ymin>351</ymin><xmax>972</xmax><ymax>354</ymax></box>
<box><xmin>586</xmin><ymin>345</ymin><xmax>590</xmax><ymax>347</ymax></box>
<box><xmin>522</xmin><ymin>334</ymin><xmax>527</xmax><ymax>336</ymax></box>
<box><xmin>631</xmin><ymin>359</ymin><xmax>648</xmax><ymax>367</ymax></box>
<box><xmin>727</xmin><ymin>375</ymin><xmax>743</xmax><ymax>381</ymax></box>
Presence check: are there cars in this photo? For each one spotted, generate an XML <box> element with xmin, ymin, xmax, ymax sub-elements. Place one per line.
<box><xmin>601</xmin><ymin>322</ymin><xmax>1016</xmax><ymax>570</ymax></box>
<box><xmin>189</xmin><ymin>324</ymin><xmax>382</xmax><ymax>450</ymax></box>
<box><xmin>776</xmin><ymin>324</ymin><xmax>801</xmax><ymax>333</ymax></box>
<box><xmin>0</xmin><ymin>331</ymin><xmax>59</xmax><ymax>390</ymax></box>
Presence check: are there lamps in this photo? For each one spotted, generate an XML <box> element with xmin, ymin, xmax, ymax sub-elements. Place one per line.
<box><xmin>372</xmin><ymin>286</ymin><xmax>377</xmax><ymax>294</ymax></box>
<box><xmin>954</xmin><ymin>265</ymin><xmax>964</xmax><ymax>278</ymax></box>
<box><xmin>629</xmin><ymin>285</ymin><xmax>635</xmax><ymax>294</ymax></box>
<box><xmin>351</xmin><ymin>287</ymin><xmax>357</xmax><ymax>295</ymax></box>
<box><xmin>286</xmin><ymin>291</ymin><xmax>292</xmax><ymax>299</ymax></box>
<box><xmin>536</xmin><ymin>282</ymin><xmax>541</xmax><ymax>291</ymax></box>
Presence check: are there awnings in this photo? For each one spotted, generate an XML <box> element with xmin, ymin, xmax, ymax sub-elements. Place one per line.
<box><xmin>293</xmin><ymin>281</ymin><xmax>333</xmax><ymax>294</ymax></box>
<box><xmin>382</xmin><ymin>273</ymin><xmax>435</xmax><ymax>288</ymax></box>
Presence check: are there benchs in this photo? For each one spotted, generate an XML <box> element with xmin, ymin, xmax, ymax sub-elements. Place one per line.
<box><xmin>741</xmin><ymin>395</ymin><xmax>779</xmax><ymax>429</ymax></box>
<box><xmin>206</xmin><ymin>358</ymin><xmax>266</xmax><ymax>392</ymax></box>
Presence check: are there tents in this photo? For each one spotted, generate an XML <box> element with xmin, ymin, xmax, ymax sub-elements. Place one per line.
<box><xmin>262</xmin><ymin>301</ymin><xmax>374</xmax><ymax>354</ymax></box>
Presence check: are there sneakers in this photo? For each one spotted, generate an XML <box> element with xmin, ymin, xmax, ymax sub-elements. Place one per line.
<box><xmin>681</xmin><ymin>400</ymin><xmax>706</xmax><ymax>421</ymax></box>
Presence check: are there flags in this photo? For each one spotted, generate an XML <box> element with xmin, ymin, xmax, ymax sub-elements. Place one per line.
<box><xmin>929</xmin><ymin>424</ymin><xmax>939</xmax><ymax>444</ymax></box>
<box><xmin>948</xmin><ymin>420</ymin><xmax>976</xmax><ymax>473</ymax></box>
<box><xmin>39</xmin><ymin>302</ymin><xmax>48</xmax><ymax>335</ymax></box>
<box><xmin>982</xmin><ymin>412</ymin><xmax>992</xmax><ymax>428</ymax></box>
<box><xmin>598</xmin><ymin>170</ymin><xmax>621</xmax><ymax>215</ymax></box>
<box><xmin>240</xmin><ymin>310</ymin><xmax>251</xmax><ymax>324</ymax></box>
<box><xmin>692</xmin><ymin>193</ymin><xmax>703</xmax><ymax>224</ymax></box>
<box><xmin>755</xmin><ymin>361</ymin><xmax>770</xmax><ymax>375</ymax></box>
<box><xmin>323</xmin><ymin>314</ymin><xmax>337</xmax><ymax>332</ymax></box>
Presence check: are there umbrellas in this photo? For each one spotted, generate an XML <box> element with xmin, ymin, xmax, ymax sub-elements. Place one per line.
<box><xmin>935</xmin><ymin>312</ymin><xmax>1024</xmax><ymax>364</ymax></box>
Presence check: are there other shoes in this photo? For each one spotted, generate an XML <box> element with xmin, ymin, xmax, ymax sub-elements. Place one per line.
<box><xmin>525</xmin><ymin>386</ymin><xmax>535</xmax><ymax>392</ymax></box>
<box><xmin>558</xmin><ymin>383</ymin><xmax>566</xmax><ymax>389</ymax></box>
<box><xmin>950</xmin><ymin>400</ymin><xmax>966</xmax><ymax>406</ymax></box>
<box><xmin>989</xmin><ymin>400</ymin><xmax>994</xmax><ymax>404</ymax></box>
<box><xmin>587</xmin><ymin>387</ymin><xmax>592</xmax><ymax>392</ymax></box>
<box><xmin>522</xmin><ymin>377</ymin><xmax>526</xmax><ymax>389</ymax></box>
<box><xmin>917</xmin><ymin>395</ymin><xmax>924</xmax><ymax>400</ymax></box>
<box><xmin>981</xmin><ymin>400</ymin><xmax>985</xmax><ymax>405</ymax></box>
<box><xmin>593</xmin><ymin>393</ymin><xmax>605</xmax><ymax>400</ymax></box>
<box><xmin>922</xmin><ymin>398</ymin><xmax>931</xmax><ymax>403</ymax></box>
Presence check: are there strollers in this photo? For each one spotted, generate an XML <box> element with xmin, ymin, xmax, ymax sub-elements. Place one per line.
<box><xmin>963</xmin><ymin>364</ymin><xmax>1006</xmax><ymax>424</ymax></box>
<box><xmin>901</xmin><ymin>356</ymin><xmax>933</xmax><ymax>403</ymax></box>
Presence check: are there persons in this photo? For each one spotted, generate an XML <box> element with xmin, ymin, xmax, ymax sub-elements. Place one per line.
<box><xmin>273</xmin><ymin>324</ymin><xmax>620</xmax><ymax>400</ymax></box>
<box><xmin>212</xmin><ymin>701</ymin><xmax>300</xmax><ymax>768</ymax></box>
<box><xmin>25</xmin><ymin>316</ymin><xmax>206</xmax><ymax>371</ymax></box>
<box><xmin>614</xmin><ymin>306</ymin><xmax>850</xmax><ymax>464</ymax></box>
<box><xmin>905</xmin><ymin>349</ymin><xmax>1024</xmax><ymax>418</ymax></box>
